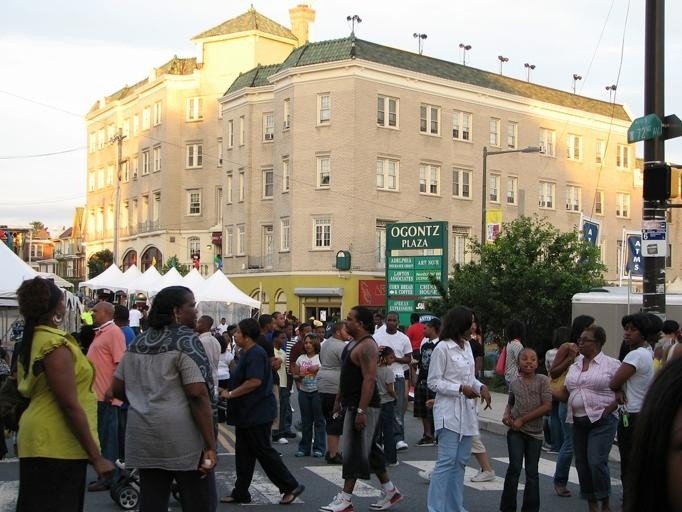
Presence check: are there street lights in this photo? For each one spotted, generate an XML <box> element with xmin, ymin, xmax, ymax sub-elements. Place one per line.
<box><xmin>479</xmin><ymin>144</ymin><xmax>542</xmax><ymax>264</ymax></box>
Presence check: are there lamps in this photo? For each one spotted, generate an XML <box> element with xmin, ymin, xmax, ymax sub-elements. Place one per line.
<box><xmin>523</xmin><ymin>63</ymin><xmax>536</xmax><ymax>84</ymax></box>
<box><xmin>604</xmin><ymin>84</ymin><xmax>616</xmax><ymax>103</ymax></box>
<box><xmin>496</xmin><ymin>55</ymin><xmax>508</xmax><ymax>76</ymax></box>
<box><xmin>459</xmin><ymin>44</ymin><xmax>471</xmax><ymax>67</ymax></box>
<box><xmin>346</xmin><ymin>15</ymin><xmax>363</xmax><ymax>37</ymax></box>
<box><xmin>569</xmin><ymin>72</ymin><xmax>583</xmax><ymax>95</ymax></box>
<box><xmin>413</xmin><ymin>32</ymin><xmax>427</xmax><ymax>56</ymax></box>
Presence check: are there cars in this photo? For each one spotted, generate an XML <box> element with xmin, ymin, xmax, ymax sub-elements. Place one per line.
<box><xmin>78</xmin><ymin>293</ymin><xmax>115</xmax><ymax>313</ymax></box>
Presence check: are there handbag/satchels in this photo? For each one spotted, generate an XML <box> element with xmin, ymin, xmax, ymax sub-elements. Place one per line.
<box><xmin>548</xmin><ymin>364</ymin><xmax>570</xmax><ymax>403</ymax></box>
<box><xmin>496</xmin><ymin>346</ymin><xmax>506</xmax><ymax>376</ymax></box>
<box><xmin>0</xmin><ymin>376</ymin><xmax>31</xmax><ymax>432</ymax></box>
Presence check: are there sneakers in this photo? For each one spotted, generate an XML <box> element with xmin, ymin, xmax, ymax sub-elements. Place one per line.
<box><xmin>369</xmin><ymin>484</ymin><xmax>405</xmax><ymax>511</ymax></box>
<box><xmin>325</xmin><ymin>451</ymin><xmax>344</xmax><ymax>464</ymax></box>
<box><xmin>295</xmin><ymin>451</ymin><xmax>305</xmax><ymax>457</ymax></box>
<box><xmin>418</xmin><ymin>470</ymin><xmax>433</xmax><ymax>481</ymax></box>
<box><xmin>471</xmin><ymin>467</ymin><xmax>496</xmax><ymax>482</ymax></box>
<box><xmin>272</xmin><ymin>436</ymin><xmax>289</xmax><ymax>444</ymax></box>
<box><xmin>554</xmin><ymin>483</ymin><xmax>570</xmax><ymax>497</ymax></box>
<box><xmin>416</xmin><ymin>435</ymin><xmax>435</xmax><ymax>447</ymax></box>
<box><xmin>396</xmin><ymin>441</ymin><xmax>408</xmax><ymax>451</ymax></box>
<box><xmin>313</xmin><ymin>451</ymin><xmax>323</xmax><ymax>457</ymax></box>
<box><xmin>318</xmin><ymin>493</ymin><xmax>354</xmax><ymax>512</ymax></box>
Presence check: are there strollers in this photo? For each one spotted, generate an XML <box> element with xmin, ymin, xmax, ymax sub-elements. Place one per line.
<box><xmin>110</xmin><ymin>405</ymin><xmax>221</xmax><ymax>510</ymax></box>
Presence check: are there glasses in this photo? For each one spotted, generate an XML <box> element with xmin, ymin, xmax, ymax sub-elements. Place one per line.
<box><xmin>576</xmin><ymin>338</ymin><xmax>598</xmax><ymax>344</ymax></box>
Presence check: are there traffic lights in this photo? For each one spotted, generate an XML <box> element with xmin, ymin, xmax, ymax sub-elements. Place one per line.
<box><xmin>640</xmin><ymin>162</ymin><xmax>680</xmax><ymax>201</ymax></box>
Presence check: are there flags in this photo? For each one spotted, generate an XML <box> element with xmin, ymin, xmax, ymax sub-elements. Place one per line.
<box><xmin>119</xmin><ymin>252</ymin><xmax>225</xmax><ymax>271</ymax></box>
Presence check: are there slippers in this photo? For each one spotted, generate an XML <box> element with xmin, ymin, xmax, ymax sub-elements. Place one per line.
<box><xmin>220</xmin><ymin>496</ymin><xmax>251</xmax><ymax>503</ymax></box>
<box><xmin>280</xmin><ymin>484</ymin><xmax>305</xmax><ymax>504</ymax></box>
